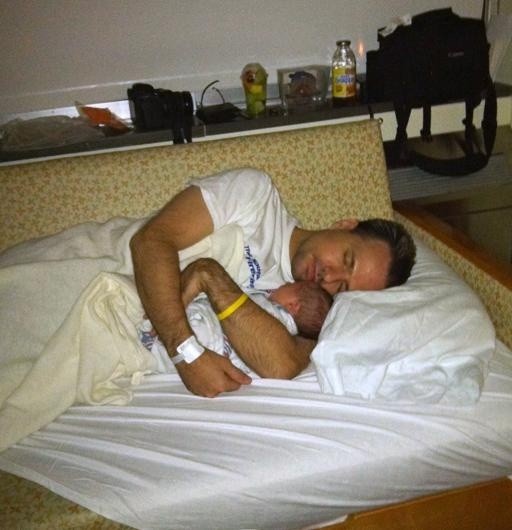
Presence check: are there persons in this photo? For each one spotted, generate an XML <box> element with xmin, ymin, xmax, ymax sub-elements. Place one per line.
<box><xmin>0</xmin><ymin>165</ymin><xmax>415</xmax><ymax>419</ymax></box>
<box><xmin>133</xmin><ymin>280</ymin><xmax>334</xmax><ymax>366</ymax></box>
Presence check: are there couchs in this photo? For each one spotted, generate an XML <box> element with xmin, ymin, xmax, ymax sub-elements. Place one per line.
<box><xmin>0</xmin><ymin>114</ymin><xmax>511</xmax><ymax>529</ymax></box>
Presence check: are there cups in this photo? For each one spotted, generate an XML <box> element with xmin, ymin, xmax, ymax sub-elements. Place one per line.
<box><xmin>240</xmin><ymin>63</ymin><xmax>268</xmax><ymax>120</ymax></box>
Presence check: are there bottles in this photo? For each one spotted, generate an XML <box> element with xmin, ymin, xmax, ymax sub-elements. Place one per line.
<box><xmin>332</xmin><ymin>41</ymin><xmax>357</xmax><ymax>108</ymax></box>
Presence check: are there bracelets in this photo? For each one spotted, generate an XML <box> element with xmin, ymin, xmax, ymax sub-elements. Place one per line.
<box><xmin>171</xmin><ymin>335</ymin><xmax>202</xmax><ymax>371</ymax></box>
<box><xmin>214</xmin><ymin>290</ymin><xmax>250</xmax><ymax>321</ymax></box>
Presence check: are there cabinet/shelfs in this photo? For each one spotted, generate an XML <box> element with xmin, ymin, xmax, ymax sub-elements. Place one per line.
<box><xmin>0</xmin><ymin>80</ymin><xmax>512</xmax><ymax>207</ymax></box>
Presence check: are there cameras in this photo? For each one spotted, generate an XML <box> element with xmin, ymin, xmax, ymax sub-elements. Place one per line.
<box><xmin>127</xmin><ymin>83</ymin><xmax>193</xmax><ymax>131</ymax></box>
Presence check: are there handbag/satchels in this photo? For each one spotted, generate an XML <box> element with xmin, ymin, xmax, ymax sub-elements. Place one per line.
<box><xmin>363</xmin><ymin>7</ymin><xmax>493</xmax><ymax>104</ymax></box>
